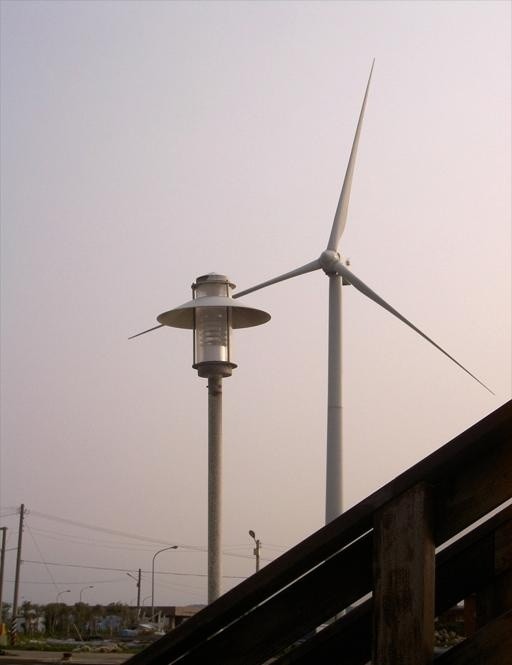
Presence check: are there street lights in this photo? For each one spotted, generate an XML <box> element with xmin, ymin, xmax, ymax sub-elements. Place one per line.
<box><xmin>54</xmin><ymin>585</ymin><xmax>95</xmax><ymax>627</ymax></box>
<box><xmin>150</xmin><ymin>543</ymin><xmax>178</xmax><ymax>625</ymax></box>
<box><xmin>156</xmin><ymin>272</ymin><xmax>273</xmax><ymax>615</ymax></box>
<box><xmin>248</xmin><ymin>529</ymin><xmax>260</xmax><ymax>574</ymax></box>
<box><xmin>126</xmin><ymin>569</ymin><xmax>141</xmax><ymax>619</ymax></box>
